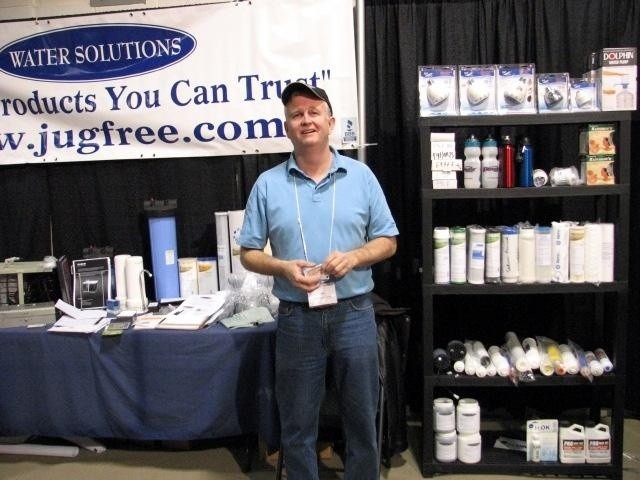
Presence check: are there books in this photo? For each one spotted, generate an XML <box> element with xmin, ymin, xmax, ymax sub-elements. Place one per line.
<box><xmin>155</xmin><ymin>287</ymin><xmax>231</xmax><ymax>331</ymax></box>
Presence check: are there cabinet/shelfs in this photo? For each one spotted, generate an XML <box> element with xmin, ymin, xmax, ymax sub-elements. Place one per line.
<box><xmin>404</xmin><ymin>109</ymin><xmax>632</xmax><ymax>480</ymax></box>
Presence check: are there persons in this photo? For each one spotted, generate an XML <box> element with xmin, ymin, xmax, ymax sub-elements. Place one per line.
<box><xmin>587</xmin><ymin>170</ymin><xmax>599</xmax><ymax>184</ymax></box>
<box><xmin>587</xmin><ymin>139</ymin><xmax>601</xmax><ymax>153</ymax></box>
<box><xmin>600</xmin><ymin>137</ymin><xmax>614</xmax><ymax>151</ymax></box>
<box><xmin>238</xmin><ymin>88</ymin><xmax>397</xmax><ymax>478</ymax></box>
<box><xmin>599</xmin><ymin>168</ymin><xmax>611</xmax><ymax>182</ymax></box>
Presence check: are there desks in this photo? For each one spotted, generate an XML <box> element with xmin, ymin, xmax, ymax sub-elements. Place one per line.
<box><xmin>1</xmin><ymin>293</ymin><xmax>411</xmax><ymax>468</ymax></box>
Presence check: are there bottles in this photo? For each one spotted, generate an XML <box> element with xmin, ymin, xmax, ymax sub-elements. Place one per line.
<box><xmin>531</xmin><ymin>433</ymin><xmax>541</xmax><ymax>463</ymax></box>
<box><xmin>481</xmin><ymin>132</ymin><xmax>500</xmax><ymax>190</ymax></box>
<box><xmin>495</xmin><ymin>137</ymin><xmax>518</xmax><ymax>189</ymax></box>
<box><xmin>463</xmin><ymin>134</ymin><xmax>480</xmax><ymax>192</ymax></box>
<box><xmin>515</xmin><ymin>135</ymin><xmax>535</xmax><ymax>187</ymax></box>
<box><xmin>434</xmin><ymin>397</ymin><xmax>482</xmax><ymax>463</ymax></box>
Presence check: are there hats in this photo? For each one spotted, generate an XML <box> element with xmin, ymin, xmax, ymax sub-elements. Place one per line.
<box><xmin>281</xmin><ymin>82</ymin><xmax>332</xmax><ymax>115</ymax></box>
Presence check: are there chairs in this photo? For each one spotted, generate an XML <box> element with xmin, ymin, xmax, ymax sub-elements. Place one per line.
<box><xmin>274</xmin><ymin>368</ymin><xmax>413</xmax><ymax>480</ymax></box>
